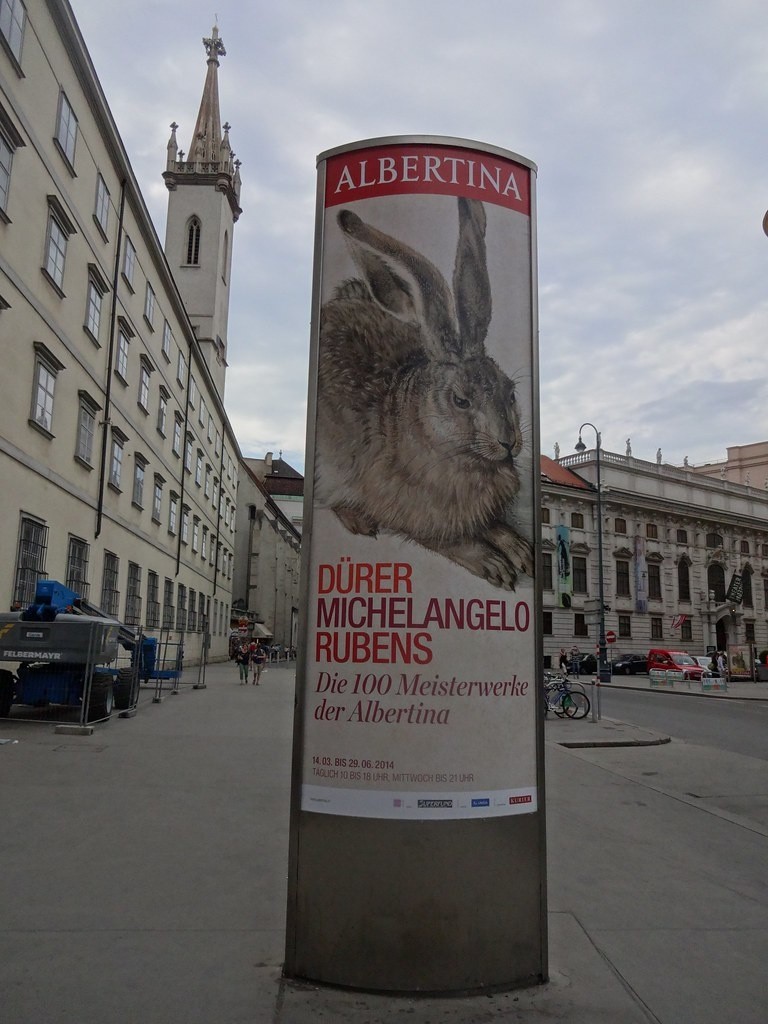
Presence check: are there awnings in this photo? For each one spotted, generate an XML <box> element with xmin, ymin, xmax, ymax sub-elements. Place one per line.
<box><xmin>251</xmin><ymin>623</ymin><xmax>274</xmax><ymax>639</ymax></box>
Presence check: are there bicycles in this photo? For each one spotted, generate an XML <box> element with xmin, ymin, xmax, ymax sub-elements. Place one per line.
<box><xmin>544</xmin><ymin>672</ymin><xmax>591</xmax><ymax>719</ymax></box>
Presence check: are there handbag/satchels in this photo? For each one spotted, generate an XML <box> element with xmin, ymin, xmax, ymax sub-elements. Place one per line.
<box><xmin>708</xmin><ymin>662</ymin><xmax>714</xmax><ymax>670</ymax></box>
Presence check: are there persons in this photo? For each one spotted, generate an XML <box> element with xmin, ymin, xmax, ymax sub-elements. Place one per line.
<box><xmin>570</xmin><ymin>645</ymin><xmax>580</xmax><ymax>679</ymax></box>
<box><xmin>236</xmin><ymin>642</ymin><xmax>290</xmax><ymax>685</ymax></box>
<box><xmin>711</xmin><ymin>651</ymin><xmax>730</xmax><ymax>688</ymax></box>
<box><xmin>559</xmin><ymin>648</ymin><xmax>569</xmax><ymax>675</ymax></box>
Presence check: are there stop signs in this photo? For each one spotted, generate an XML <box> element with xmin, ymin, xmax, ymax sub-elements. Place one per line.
<box><xmin>606</xmin><ymin>631</ymin><xmax>616</xmax><ymax>643</ymax></box>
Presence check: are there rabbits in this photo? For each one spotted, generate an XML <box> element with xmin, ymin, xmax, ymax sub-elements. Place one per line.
<box><xmin>310</xmin><ymin>197</ymin><xmax>539</xmax><ymax>594</ymax></box>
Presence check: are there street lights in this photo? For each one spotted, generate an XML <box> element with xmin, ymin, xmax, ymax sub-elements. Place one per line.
<box><xmin>573</xmin><ymin>421</ymin><xmax>613</xmax><ymax>684</ymax></box>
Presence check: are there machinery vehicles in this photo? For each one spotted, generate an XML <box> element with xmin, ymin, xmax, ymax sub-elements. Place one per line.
<box><xmin>0</xmin><ymin>578</ymin><xmax>184</xmax><ymax>724</ymax></box>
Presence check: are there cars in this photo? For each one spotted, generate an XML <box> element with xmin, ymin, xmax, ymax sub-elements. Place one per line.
<box><xmin>677</xmin><ymin>655</ymin><xmax>724</xmax><ymax>678</ymax></box>
<box><xmin>566</xmin><ymin>652</ymin><xmax>597</xmax><ymax>674</ymax></box>
<box><xmin>647</xmin><ymin>648</ymin><xmax>707</xmax><ymax>681</ymax></box>
<box><xmin>609</xmin><ymin>653</ymin><xmax>655</xmax><ymax>675</ymax></box>
